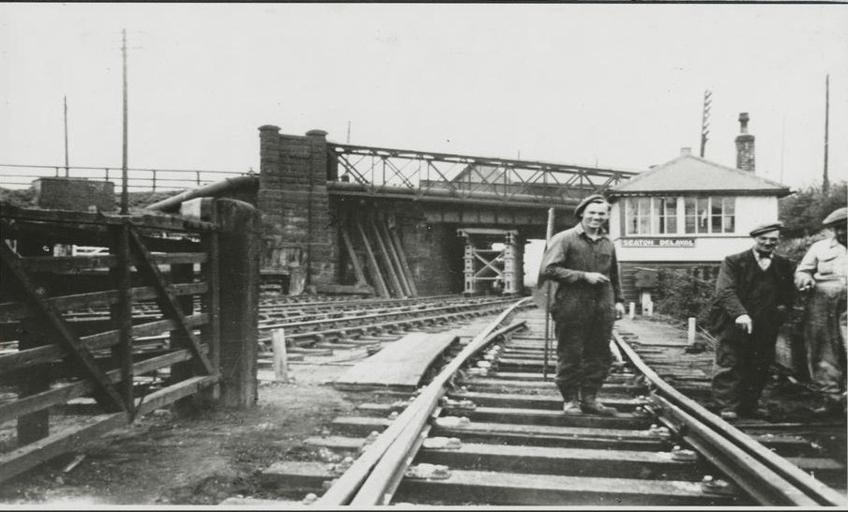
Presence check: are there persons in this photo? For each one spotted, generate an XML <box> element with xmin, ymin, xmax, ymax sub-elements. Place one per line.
<box><xmin>708</xmin><ymin>214</ymin><xmax>796</xmax><ymax>420</ymax></box>
<box><xmin>539</xmin><ymin>192</ymin><xmax>626</xmax><ymax>420</ymax></box>
<box><xmin>791</xmin><ymin>208</ymin><xmax>848</xmax><ymax>419</ymax></box>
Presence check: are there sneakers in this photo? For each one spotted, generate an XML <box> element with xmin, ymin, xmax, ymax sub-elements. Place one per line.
<box><xmin>563</xmin><ymin>400</ymin><xmax>583</xmax><ymax>417</ymax></box>
<box><xmin>580</xmin><ymin>398</ymin><xmax>617</xmax><ymax>416</ymax></box>
<box><xmin>721</xmin><ymin>403</ymin><xmax>772</xmax><ymax>421</ymax></box>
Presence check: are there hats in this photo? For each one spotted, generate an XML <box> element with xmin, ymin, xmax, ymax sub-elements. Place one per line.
<box><xmin>822</xmin><ymin>207</ymin><xmax>848</xmax><ymax>227</ymax></box>
<box><xmin>574</xmin><ymin>194</ymin><xmax>605</xmax><ymax>220</ymax></box>
<box><xmin>749</xmin><ymin>220</ymin><xmax>784</xmax><ymax>239</ymax></box>
